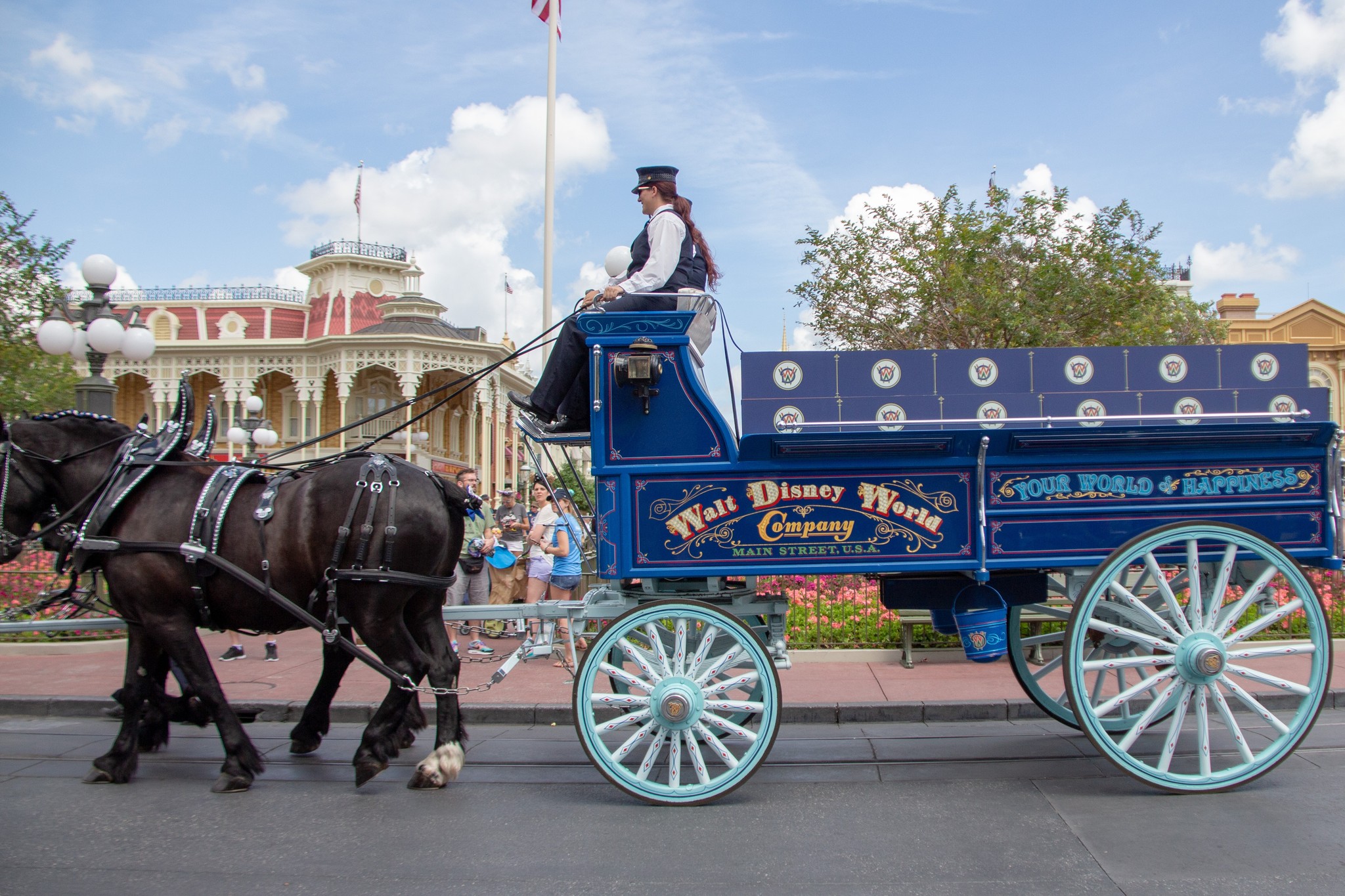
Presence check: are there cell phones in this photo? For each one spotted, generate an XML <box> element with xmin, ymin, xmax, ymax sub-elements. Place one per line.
<box><xmin>505</xmin><ymin>524</ymin><xmax>510</xmax><ymax>527</ymax></box>
<box><xmin>529</xmin><ymin>538</ymin><xmax>540</xmax><ymax>544</ymax></box>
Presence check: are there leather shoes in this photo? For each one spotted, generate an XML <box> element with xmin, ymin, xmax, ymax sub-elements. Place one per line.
<box><xmin>544</xmin><ymin>418</ymin><xmax>591</xmax><ymax>433</ymax></box>
<box><xmin>507</xmin><ymin>390</ymin><xmax>554</xmax><ymax>423</ymax></box>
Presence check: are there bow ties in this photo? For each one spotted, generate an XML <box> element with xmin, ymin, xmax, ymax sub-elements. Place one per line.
<box><xmin>465</xmin><ymin>508</ymin><xmax>485</xmax><ymax>522</ymax></box>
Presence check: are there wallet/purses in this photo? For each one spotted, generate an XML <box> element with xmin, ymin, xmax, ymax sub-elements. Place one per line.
<box><xmin>458</xmin><ymin>555</ymin><xmax>484</xmax><ymax>574</ymax></box>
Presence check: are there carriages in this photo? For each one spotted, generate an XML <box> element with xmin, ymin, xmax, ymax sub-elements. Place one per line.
<box><xmin>0</xmin><ymin>277</ymin><xmax>1341</xmax><ymax>808</ymax></box>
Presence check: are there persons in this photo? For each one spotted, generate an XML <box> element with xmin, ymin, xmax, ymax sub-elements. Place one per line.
<box><xmin>507</xmin><ymin>165</ymin><xmax>724</xmax><ymax>432</ymax></box>
<box><xmin>442</xmin><ymin>468</ymin><xmax>588</xmax><ymax>669</ymax></box>
<box><xmin>218</xmin><ymin>629</ymin><xmax>280</xmax><ymax>662</ymax></box>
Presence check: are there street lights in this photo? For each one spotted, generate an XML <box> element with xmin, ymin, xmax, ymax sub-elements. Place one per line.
<box><xmin>225</xmin><ymin>394</ymin><xmax>279</xmax><ymax>471</ymax></box>
<box><xmin>35</xmin><ymin>253</ymin><xmax>157</xmax><ymax>418</ymax></box>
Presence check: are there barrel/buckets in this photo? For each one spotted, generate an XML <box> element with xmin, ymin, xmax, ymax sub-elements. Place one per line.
<box><xmin>929</xmin><ymin>609</ymin><xmax>969</xmax><ymax>636</ymax></box>
<box><xmin>951</xmin><ymin>583</ymin><xmax>1007</xmax><ymax>663</ymax></box>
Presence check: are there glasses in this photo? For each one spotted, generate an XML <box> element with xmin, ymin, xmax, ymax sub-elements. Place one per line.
<box><xmin>462</xmin><ymin>478</ymin><xmax>477</xmax><ymax>482</ymax></box>
<box><xmin>637</xmin><ymin>186</ymin><xmax>651</xmax><ymax>197</ymax></box>
<box><xmin>501</xmin><ymin>500</ymin><xmax>512</xmax><ymax>504</ymax></box>
<box><xmin>533</xmin><ymin>506</ymin><xmax>535</xmax><ymax>508</ymax></box>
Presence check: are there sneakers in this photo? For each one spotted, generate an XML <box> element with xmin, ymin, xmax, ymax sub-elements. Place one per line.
<box><xmin>449</xmin><ymin>640</ymin><xmax>462</xmax><ymax>660</ymax></box>
<box><xmin>467</xmin><ymin>639</ymin><xmax>495</xmax><ymax>655</ymax></box>
<box><xmin>219</xmin><ymin>644</ymin><xmax>245</xmax><ymax>661</ymax></box>
<box><xmin>265</xmin><ymin>639</ymin><xmax>280</xmax><ymax>661</ymax></box>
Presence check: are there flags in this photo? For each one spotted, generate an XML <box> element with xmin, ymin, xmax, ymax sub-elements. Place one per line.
<box><xmin>531</xmin><ymin>0</ymin><xmax>562</xmax><ymax>43</ymax></box>
<box><xmin>354</xmin><ymin>165</ymin><xmax>363</xmax><ymax>214</ymax></box>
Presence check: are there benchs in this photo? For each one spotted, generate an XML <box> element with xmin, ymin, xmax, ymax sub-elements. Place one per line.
<box><xmin>576</xmin><ymin>285</ymin><xmax>719</xmax><ymax>358</ymax></box>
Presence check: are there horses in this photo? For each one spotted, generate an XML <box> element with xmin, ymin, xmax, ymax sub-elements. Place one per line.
<box><xmin>23</xmin><ymin>410</ymin><xmax>483</xmax><ymax>753</ymax></box>
<box><xmin>0</xmin><ymin>413</ymin><xmax>470</xmax><ymax>792</ymax></box>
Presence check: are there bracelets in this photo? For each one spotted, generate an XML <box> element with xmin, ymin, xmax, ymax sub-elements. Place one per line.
<box><xmin>544</xmin><ymin>546</ymin><xmax>551</xmax><ymax>554</ymax></box>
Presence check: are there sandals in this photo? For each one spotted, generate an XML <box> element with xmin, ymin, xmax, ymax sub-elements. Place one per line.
<box><xmin>575</xmin><ymin>640</ymin><xmax>587</xmax><ymax>651</ymax></box>
<box><xmin>554</xmin><ymin>658</ymin><xmax>574</xmax><ymax>667</ymax></box>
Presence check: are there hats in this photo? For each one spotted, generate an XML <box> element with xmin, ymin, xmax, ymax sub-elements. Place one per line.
<box><xmin>673</xmin><ymin>195</ymin><xmax>693</xmax><ymax>217</ymax></box>
<box><xmin>631</xmin><ymin>165</ymin><xmax>679</xmax><ymax>194</ymax></box>
<box><xmin>531</xmin><ymin>501</ymin><xmax>539</xmax><ymax>508</ymax></box>
<box><xmin>546</xmin><ymin>489</ymin><xmax>571</xmax><ymax>502</ymax></box>
<box><xmin>481</xmin><ymin>494</ymin><xmax>489</xmax><ymax>499</ymax></box>
